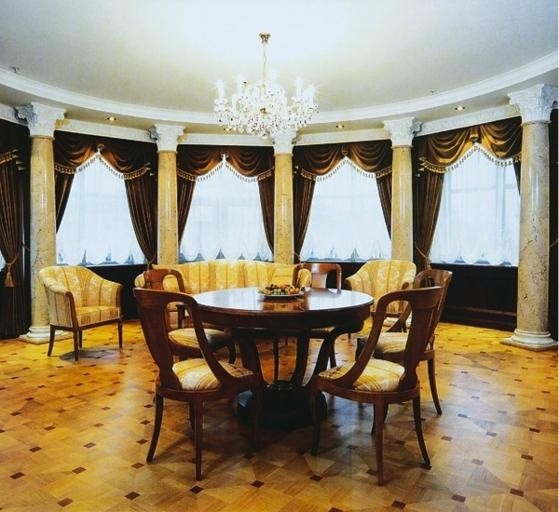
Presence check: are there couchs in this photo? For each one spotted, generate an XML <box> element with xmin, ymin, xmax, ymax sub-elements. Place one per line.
<box><xmin>132</xmin><ymin>260</ymin><xmax>454</xmax><ymax>485</ymax></box>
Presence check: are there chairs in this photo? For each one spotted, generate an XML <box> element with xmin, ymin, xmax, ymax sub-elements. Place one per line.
<box><xmin>40</xmin><ymin>266</ymin><xmax>124</xmax><ymax>360</ymax></box>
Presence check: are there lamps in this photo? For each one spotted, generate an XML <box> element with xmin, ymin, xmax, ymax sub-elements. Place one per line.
<box><xmin>214</xmin><ymin>32</ymin><xmax>318</xmax><ymax>142</ymax></box>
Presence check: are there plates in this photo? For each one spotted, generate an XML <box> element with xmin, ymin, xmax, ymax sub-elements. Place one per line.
<box><xmin>255</xmin><ymin>290</ymin><xmax>304</xmax><ymax>298</ymax></box>
